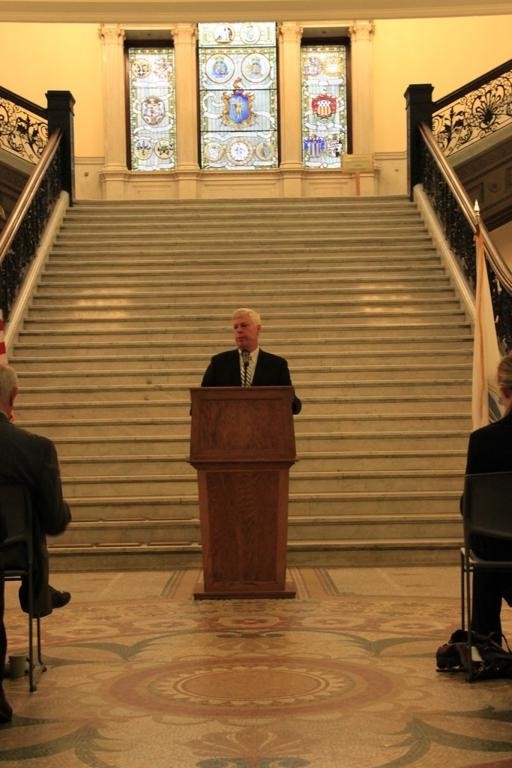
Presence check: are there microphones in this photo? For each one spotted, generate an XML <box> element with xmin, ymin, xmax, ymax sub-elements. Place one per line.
<box><xmin>242</xmin><ymin>349</ymin><xmax>250</xmax><ymax>366</ymax></box>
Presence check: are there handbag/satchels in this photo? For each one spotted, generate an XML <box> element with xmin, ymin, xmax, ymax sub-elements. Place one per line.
<box><xmin>437</xmin><ymin>630</ymin><xmax>512</xmax><ymax>681</ymax></box>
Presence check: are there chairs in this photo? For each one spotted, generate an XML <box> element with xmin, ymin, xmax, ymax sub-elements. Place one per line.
<box><xmin>2</xmin><ymin>466</ymin><xmax>47</xmax><ymax>695</ymax></box>
<box><xmin>457</xmin><ymin>468</ymin><xmax>512</xmax><ymax>680</ymax></box>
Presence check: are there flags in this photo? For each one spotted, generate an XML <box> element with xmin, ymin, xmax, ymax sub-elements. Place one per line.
<box><xmin>468</xmin><ymin>225</ymin><xmax>504</xmax><ymax>432</ymax></box>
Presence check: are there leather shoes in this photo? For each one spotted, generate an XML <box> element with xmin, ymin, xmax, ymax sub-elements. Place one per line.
<box><xmin>31</xmin><ymin>589</ymin><xmax>71</xmax><ymax>618</ymax></box>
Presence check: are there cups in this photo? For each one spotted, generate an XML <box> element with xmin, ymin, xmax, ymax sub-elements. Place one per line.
<box><xmin>9</xmin><ymin>654</ymin><xmax>27</xmax><ymax>678</ymax></box>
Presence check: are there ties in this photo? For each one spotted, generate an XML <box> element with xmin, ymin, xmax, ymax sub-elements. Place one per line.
<box><xmin>241</xmin><ymin>355</ymin><xmax>253</xmax><ymax>387</ymax></box>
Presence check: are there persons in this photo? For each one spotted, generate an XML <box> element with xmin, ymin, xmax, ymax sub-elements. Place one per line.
<box><xmin>0</xmin><ymin>365</ymin><xmax>71</xmax><ymax>617</ymax></box>
<box><xmin>461</xmin><ymin>349</ymin><xmax>512</xmax><ymax>652</ymax></box>
<box><xmin>199</xmin><ymin>307</ymin><xmax>302</xmax><ymax>415</ymax></box>
